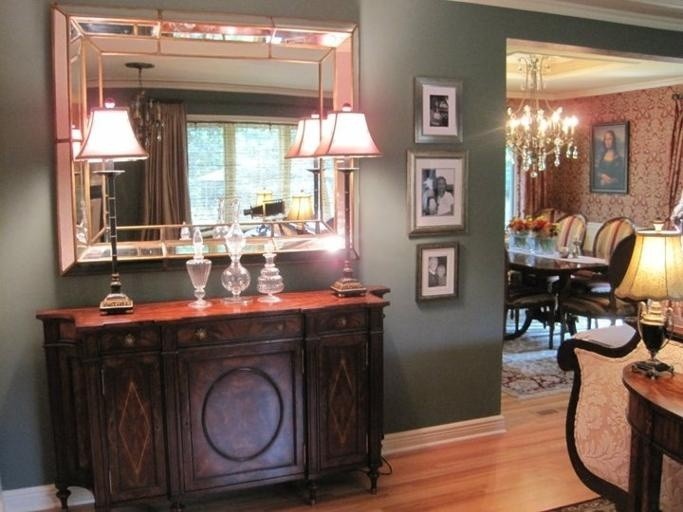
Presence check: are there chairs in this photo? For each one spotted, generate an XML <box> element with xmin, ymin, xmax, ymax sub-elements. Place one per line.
<box><xmin>535</xmin><ymin>208</ymin><xmax>553</xmax><ymax>237</ymax></box>
<box><xmin>552</xmin><ymin>214</ymin><xmax>586</xmax><ymax>255</ymax></box>
<box><xmin>548</xmin><ymin>218</ymin><xmax>635</xmax><ymax>350</ymax></box>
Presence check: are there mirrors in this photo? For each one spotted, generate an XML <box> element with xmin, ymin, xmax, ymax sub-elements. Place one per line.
<box><xmin>53</xmin><ymin>10</ymin><xmax>359</xmax><ymax>276</ymax></box>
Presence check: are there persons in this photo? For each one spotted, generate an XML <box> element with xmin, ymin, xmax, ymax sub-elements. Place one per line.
<box><xmin>431</xmin><ymin>97</ymin><xmax>446</xmax><ymax>126</ymax></box>
<box><xmin>590</xmin><ymin>131</ymin><xmax>625</xmax><ymax>192</ymax></box>
<box><xmin>429</xmin><ymin>256</ymin><xmax>439</xmax><ymax>288</ymax></box>
<box><xmin>422</xmin><ymin>174</ymin><xmax>454</xmax><ymax>215</ymax></box>
<box><xmin>437</xmin><ymin>264</ymin><xmax>447</xmax><ymax>285</ymax></box>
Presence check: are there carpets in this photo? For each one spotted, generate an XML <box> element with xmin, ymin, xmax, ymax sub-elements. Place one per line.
<box><xmin>501</xmin><ymin>308</ymin><xmax>576</xmax><ymax>399</ymax></box>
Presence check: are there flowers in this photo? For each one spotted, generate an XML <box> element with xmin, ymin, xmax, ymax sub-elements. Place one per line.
<box><xmin>507</xmin><ymin>217</ymin><xmax>533</xmax><ymax>231</ymax></box>
<box><xmin>524</xmin><ymin>216</ymin><xmax>562</xmax><ymax>236</ymax></box>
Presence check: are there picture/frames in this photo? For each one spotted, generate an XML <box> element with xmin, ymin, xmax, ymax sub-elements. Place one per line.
<box><xmin>417</xmin><ymin>242</ymin><xmax>460</xmax><ymax>301</ymax></box>
<box><xmin>408</xmin><ymin>152</ymin><xmax>468</xmax><ymax>237</ymax></box>
<box><xmin>416</xmin><ymin>76</ymin><xmax>464</xmax><ymax>143</ymax></box>
<box><xmin>589</xmin><ymin>123</ymin><xmax>629</xmax><ymax>192</ymax></box>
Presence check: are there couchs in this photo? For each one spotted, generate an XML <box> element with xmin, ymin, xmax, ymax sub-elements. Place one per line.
<box><xmin>558</xmin><ymin>324</ymin><xmax>683</xmax><ymax>512</ymax></box>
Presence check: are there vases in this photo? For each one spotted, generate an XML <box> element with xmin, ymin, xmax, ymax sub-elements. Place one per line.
<box><xmin>511</xmin><ymin>230</ymin><xmax>528</xmax><ymax>239</ymax></box>
<box><xmin>534</xmin><ymin>237</ymin><xmax>558</xmax><ymax>254</ymax></box>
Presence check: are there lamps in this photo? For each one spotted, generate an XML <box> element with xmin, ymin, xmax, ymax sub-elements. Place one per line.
<box><xmin>614</xmin><ymin>216</ymin><xmax>683</xmax><ymax>378</ymax></box>
<box><xmin>505</xmin><ymin>58</ymin><xmax>579</xmax><ymax>178</ymax></box>
<box><xmin>314</xmin><ymin>102</ymin><xmax>381</xmax><ymax>297</ymax></box>
<box><xmin>286</xmin><ymin>114</ymin><xmax>334</xmax><ymax>158</ymax></box>
<box><xmin>286</xmin><ymin>191</ymin><xmax>314</xmax><ymax>233</ymax></box>
<box><xmin>258</xmin><ymin>187</ymin><xmax>273</xmax><ymax>218</ymax></box>
<box><xmin>72</xmin><ymin>99</ymin><xmax>151</xmax><ymax>311</ymax></box>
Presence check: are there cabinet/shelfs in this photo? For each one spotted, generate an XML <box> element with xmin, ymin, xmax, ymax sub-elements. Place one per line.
<box><xmin>35</xmin><ymin>286</ymin><xmax>392</xmax><ymax>512</ymax></box>
